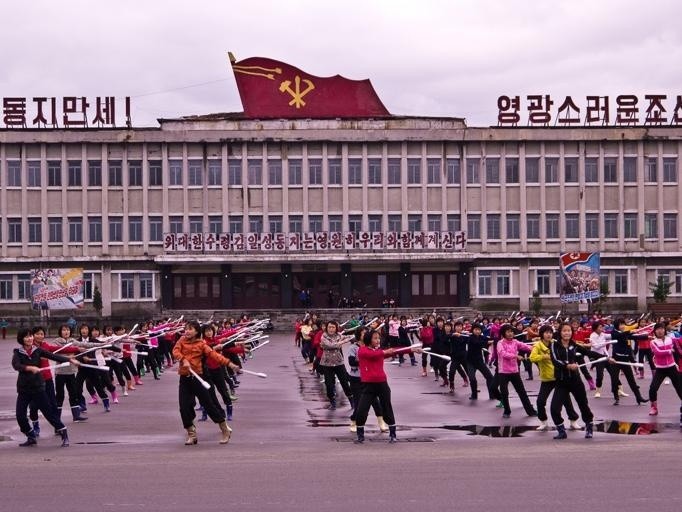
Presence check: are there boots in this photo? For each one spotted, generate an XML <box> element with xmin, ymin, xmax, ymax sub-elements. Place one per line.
<box><xmin>353</xmin><ymin>424</ymin><xmax>366</xmax><ymax>445</ymax></box>
<box><xmin>388</xmin><ymin>423</ymin><xmax>397</xmax><ymax>445</ymax></box>
<box><xmin>18</xmin><ymin>419</ymin><xmax>41</xmax><ymax>448</ymax></box>
<box><xmin>218</xmin><ymin>421</ymin><xmax>233</xmax><ymax>446</ymax></box>
<box><xmin>193</xmin><ymin>351</ymin><xmax>253</xmax><ymax>422</ymax></box>
<box><xmin>376</xmin><ymin>415</ymin><xmax>387</xmax><ymax>431</ymax></box>
<box><xmin>71</xmin><ymin>358</ymin><xmax>174</xmax><ymax>412</ymax></box>
<box><xmin>303</xmin><ymin>358</ymin><xmax>360</xmax><ymax>411</ymax></box>
<box><xmin>537</xmin><ymin>416</ymin><xmax>594</xmax><ymax>440</ymax></box>
<box><xmin>57</xmin><ymin>426</ymin><xmax>71</xmax><ymax>448</ymax></box>
<box><xmin>587</xmin><ymin>369</ymin><xmax>670</xmax><ymax>416</ymax></box>
<box><xmin>350</xmin><ymin>420</ymin><xmax>358</xmax><ymax>432</ymax></box>
<box><xmin>391</xmin><ymin>356</ymin><xmax>539</xmax><ymax>419</ymax></box>
<box><xmin>70</xmin><ymin>405</ymin><xmax>88</xmax><ymax>424</ymax></box>
<box><xmin>184</xmin><ymin>424</ymin><xmax>198</xmax><ymax>446</ymax></box>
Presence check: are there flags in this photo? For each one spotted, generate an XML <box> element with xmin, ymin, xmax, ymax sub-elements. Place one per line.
<box><xmin>561</xmin><ymin>252</ymin><xmax>594</xmax><ymax>269</ymax></box>
<box><xmin>232</xmin><ymin>57</ymin><xmax>391</xmax><ymax>118</ymax></box>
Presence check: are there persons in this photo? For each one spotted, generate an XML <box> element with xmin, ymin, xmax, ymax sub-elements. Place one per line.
<box><xmin>328</xmin><ymin>290</ymin><xmax>367</xmax><ymax>308</ymax></box>
<box><xmin>45</xmin><ymin>270</ymin><xmax>57</xmax><ymax>285</ymax></box>
<box><xmin>295</xmin><ymin>311</ymin><xmax>682</xmax><ymax>446</ymax></box>
<box><xmin>565</xmin><ymin>270</ymin><xmax>600</xmax><ymax>293</ymax></box>
<box><xmin>31</xmin><ymin>269</ymin><xmax>45</xmax><ymax>285</ymax></box>
<box><xmin>1</xmin><ymin>318</ymin><xmax>9</xmax><ymax>339</ymax></box>
<box><xmin>11</xmin><ymin>315</ymin><xmax>273</xmax><ymax>446</ymax></box>
<box><xmin>298</xmin><ymin>290</ymin><xmax>314</xmax><ymax>308</ymax></box>
<box><xmin>383</xmin><ymin>297</ymin><xmax>396</xmax><ymax>308</ymax></box>
